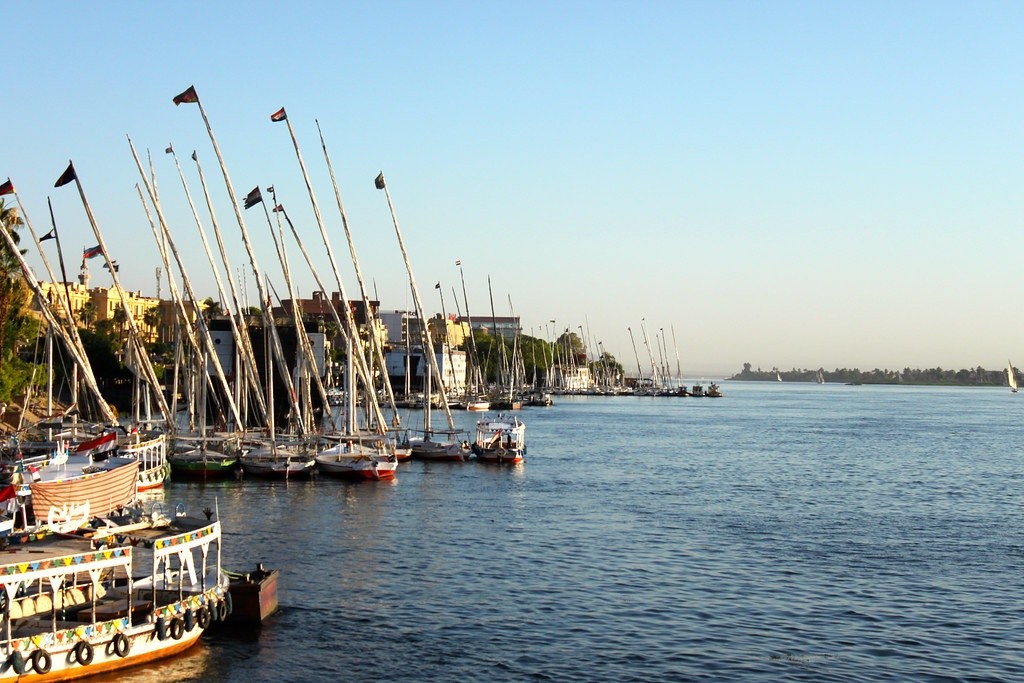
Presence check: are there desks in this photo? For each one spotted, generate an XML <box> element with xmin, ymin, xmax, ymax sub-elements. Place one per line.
<box><xmin>77</xmin><ymin>599</ymin><xmax>152</xmax><ymax>622</ymax></box>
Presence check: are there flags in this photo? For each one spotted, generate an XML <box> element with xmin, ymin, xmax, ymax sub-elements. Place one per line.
<box><xmin>273</xmin><ymin>205</ymin><xmax>283</xmax><ymax>212</ymax></box>
<box><xmin>166</xmin><ymin>148</ymin><xmax>173</xmax><ymax>153</ymax></box>
<box><xmin>267</xmin><ymin>187</ymin><xmax>274</xmax><ymax>192</ymax></box>
<box><xmin>54</xmin><ymin>165</ymin><xmax>76</xmax><ymax>188</ymax></box>
<box><xmin>375</xmin><ymin>174</ymin><xmax>385</xmax><ymax>189</ymax></box>
<box><xmin>76</xmin><ymin>433</ymin><xmax>118</xmax><ymax>457</ymax></box>
<box><xmin>244</xmin><ymin>187</ymin><xmax>262</xmax><ymax>209</ymax></box>
<box><xmin>84</xmin><ymin>245</ymin><xmax>105</xmax><ymax>259</ymax></box>
<box><xmin>455</xmin><ymin>260</ymin><xmax>461</xmax><ymax>266</ymax></box>
<box><xmin>173</xmin><ymin>86</ymin><xmax>198</xmax><ymax>106</ymax></box>
<box><xmin>435</xmin><ymin>283</ymin><xmax>440</xmax><ymax>289</ymax></box>
<box><xmin>271</xmin><ymin>108</ymin><xmax>287</xmax><ymax>122</ymax></box>
<box><xmin>0</xmin><ymin>464</ymin><xmax>25</xmax><ymax>504</ymax></box>
<box><xmin>104</xmin><ymin>260</ymin><xmax>119</xmax><ymax>272</ymax></box>
<box><xmin>0</xmin><ymin>180</ymin><xmax>15</xmax><ymax>196</ymax></box>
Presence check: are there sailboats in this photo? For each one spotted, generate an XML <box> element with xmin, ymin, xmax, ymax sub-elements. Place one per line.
<box><xmin>0</xmin><ymin>85</ymin><xmax>473</xmax><ymax>481</ymax></box>
<box><xmin>539</xmin><ymin>318</ymin><xmax>690</xmax><ymax>396</ymax></box>
<box><xmin>1008</xmin><ymin>361</ymin><xmax>1019</xmax><ymax>392</ymax></box>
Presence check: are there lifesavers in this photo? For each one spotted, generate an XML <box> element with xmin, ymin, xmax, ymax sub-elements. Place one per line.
<box><xmin>199</xmin><ymin>607</ymin><xmax>210</xmax><ymax>628</ymax></box>
<box><xmin>171</xmin><ymin>619</ymin><xmax>184</xmax><ymax>640</ymax></box>
<box><xmin>217</xmin><ymin>601</ymin><xmax>227</xmax><ymax>621</ymax></box>
<box><xmin>113</xmin><ymin>633</ymin><xmax>131</xmax><ymax>658</ymax></box>
<box><xmin>75</xmin><ymin>642</ymin><xmax>94</xmax><ymax>665</ymax></box>
<box><xmin>33</xmin><ymin>649</ymin><xmax>51</xmax><ymax>673</ymax></box>
<box><xmin>498</xmin><ymin>447</ymin><xmax>507</xmax><ymax>456</ymax></box>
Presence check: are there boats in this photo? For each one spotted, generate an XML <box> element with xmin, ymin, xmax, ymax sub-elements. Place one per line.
<box><xmin>471</xmin><ymin>415</ymin><xmax>527</xmax><ymax>464</ymax></box>
<box><xmin>705</xmin><ymin>383</ymin><xmax>723</xmax><ymax>397</ymax></box>
<box><xmin>467</xmin><ymin>400</ymin><xmax>490</xmax><ymax>412</ymax></box>
<box><xmin>689</xmin><ymin>385</ymin><xmax>706</xmax><ymax>396</ymax></box>
<box><xmin>0</xmin><ymin>495</ymin><xmax>229</xmax><ymax>683</ymax></box>
<box><xmin>0</xmin><ymin>450</ymin><xmax>144</xmax><ymax>528</ymax></box>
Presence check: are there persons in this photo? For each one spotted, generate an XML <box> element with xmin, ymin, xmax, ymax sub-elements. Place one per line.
<box><xmin>27</xmin><ymin>465</ymin><xmax>40</xmax><ymax>481</ymax></box>
